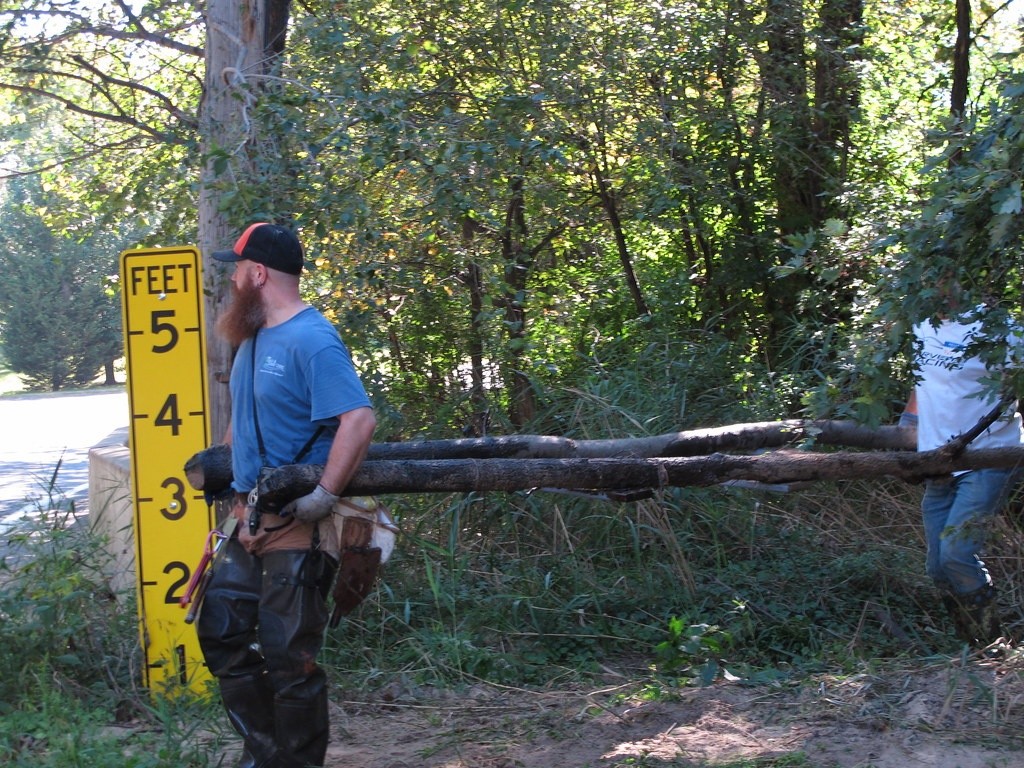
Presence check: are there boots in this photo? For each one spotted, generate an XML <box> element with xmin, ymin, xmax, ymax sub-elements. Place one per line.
<box><xmin>942</xmin><ymin>582</ymin><xmax>1007</xmax><ymax>659</ymax></box>
<box><xmin>220</xmin><ymin>664</ymin><xmax>275</xmax><ymax>768</ymax></box>
<box><xmin>273</xmin><ymin>688</ymin><xmax>329</xmax><ymax>768</ymax></box>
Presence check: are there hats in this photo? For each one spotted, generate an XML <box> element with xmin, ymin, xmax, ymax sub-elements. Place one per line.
<box><xmin>211</xmin><ymin>222</ymin><xmax>303</xmax><ymax>274</ymax></box>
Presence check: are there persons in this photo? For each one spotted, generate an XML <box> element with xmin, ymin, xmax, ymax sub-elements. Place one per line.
<box><xmin>900</xmin><ymin>271</ymin><xmax>1024</xmax><ymax>644</ymax></box>
<box><xmin>199</xmin><ymin>223</ymin><xmax>377</xmax><ymax>767</ymax></box>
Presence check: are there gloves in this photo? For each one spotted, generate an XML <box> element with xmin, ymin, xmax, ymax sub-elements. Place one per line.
<box><xmin>898</xmin><ymin>413</ymin><xmax>919</xmax><ymax>429</ymax></box>
<box><xmin>279</xmin><ymin>484</ymin><xmax>340</xmax><ymax>523</ymax></box>
